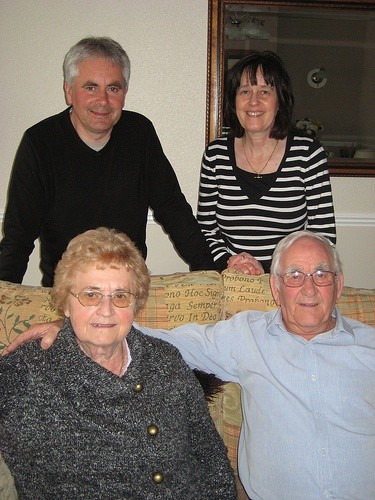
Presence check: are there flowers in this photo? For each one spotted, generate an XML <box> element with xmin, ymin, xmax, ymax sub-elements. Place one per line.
<box><xmin>296</xmin><ymin>117</ymin><xmax>327</xmax><ymax>133</ymax></box>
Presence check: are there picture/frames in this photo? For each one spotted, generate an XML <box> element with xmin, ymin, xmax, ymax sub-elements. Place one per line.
<box><xmin>206</xmin><ymin>0</ymin><xmax>375</xmax><ymax>179</ymax></box>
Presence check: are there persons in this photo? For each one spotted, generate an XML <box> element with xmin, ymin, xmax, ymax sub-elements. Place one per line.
<box><xmin>0</xmin><ymin>37</ymin><xmax>215</xmax><ymax>285</ymax></box>
<box><xmin>195</xmin><ymin>52</ymin><xmax>337</xmax><ymax>276</ymax></box>
<box><xmin>0</xmin><ymin>228</ymin><xmax>238</xmax><ymax>500</ymax></box>
<box><xmin>1</xmin><ymin>228</ymin><xmax>375</xmax><ymax>500</ymax></box>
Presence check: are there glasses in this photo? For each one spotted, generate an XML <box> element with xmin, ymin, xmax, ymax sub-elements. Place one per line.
<box><xmin>276</xmin><ymin>269</ymin><xmax>338</xmax><ymax>287</ymax></box>
<box><xmin>69</xmin><ymin>290</ymin><xmax>138</xmax><ymax>308</ymax></box>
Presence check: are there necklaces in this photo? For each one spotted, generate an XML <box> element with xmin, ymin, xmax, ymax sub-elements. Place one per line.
<box><xmin>241</xmin><ymin>136</ymin><xmax>279</xmax><ymax>179</ymax></box>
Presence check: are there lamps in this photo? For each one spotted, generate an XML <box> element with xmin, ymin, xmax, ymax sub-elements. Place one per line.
<box><xmin>223</xmin><ymin>4</ymin><xmax>272</xmax><ymax>42</ymax></box>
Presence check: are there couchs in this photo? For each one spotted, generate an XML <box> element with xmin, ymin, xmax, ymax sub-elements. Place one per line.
<box><xmin>0</xmin><ymin>267</ymin><xmax>375</xmax><ymax>500</ymax></box>
